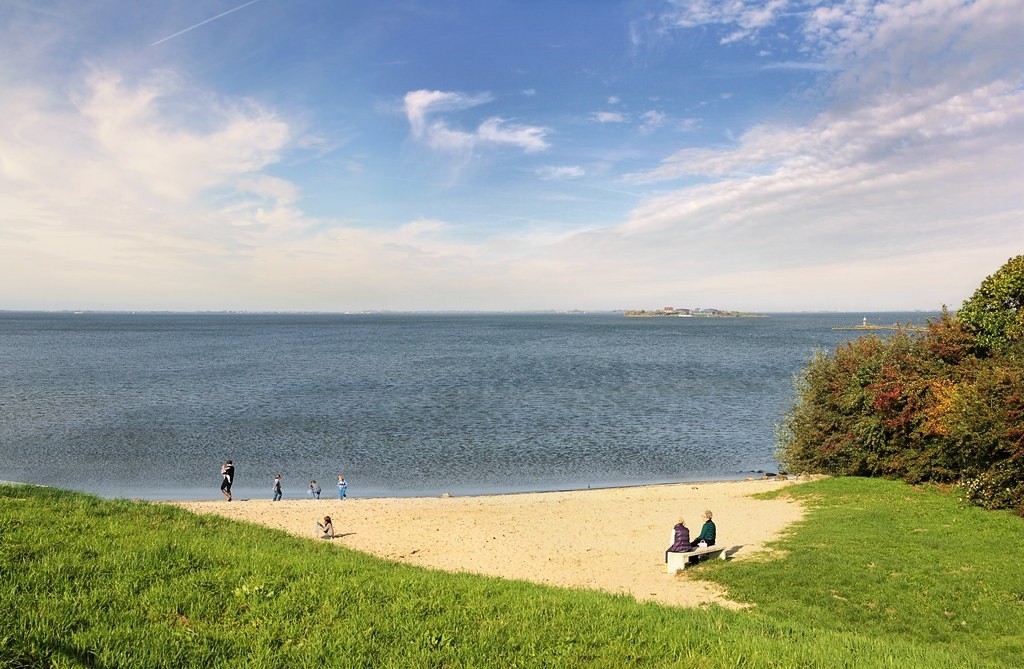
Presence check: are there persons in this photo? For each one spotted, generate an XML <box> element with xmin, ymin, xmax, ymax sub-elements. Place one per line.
<box><xmin>665</xmin><ymin>517</ymin><xmax>692</xmax><ymax>563</ymax></box>
<box><xmin>272</xmin><ymin>473</ymin><xmax>282</xmax><ymax>501</ymax></box>
<box><xmin>317</xmin><ymin>515</ymin><xmax>335</xmax><ymax>539</ymax></box>
<box><xmin>336</xmin><ymin>475</ymin><xmax>348</xmax><ymax>500</ymax></box>
<box><xmin>221</xmin><ymin>464</ymin><xmax>231</xmax><ymax>483</ymax></box>
<box><xmin>310</xmin><ymin>479</ymin><xmax>321</xmax><ymax>499</ymax></box>
<box><xmin>220</xmin><ymin>459</ymin><xmax>235</xmax><ymax>501</ymax></box>
<box><xmin>690</xmin><ymin>510</ymin><xmax>717</xmax><ymax>564</ymax></box>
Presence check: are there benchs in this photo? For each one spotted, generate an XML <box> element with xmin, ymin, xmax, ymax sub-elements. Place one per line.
<box><xmin>667</xmin><ymin>545</ymin><xmax>727</xmax><ymax>573</ymax></box>
